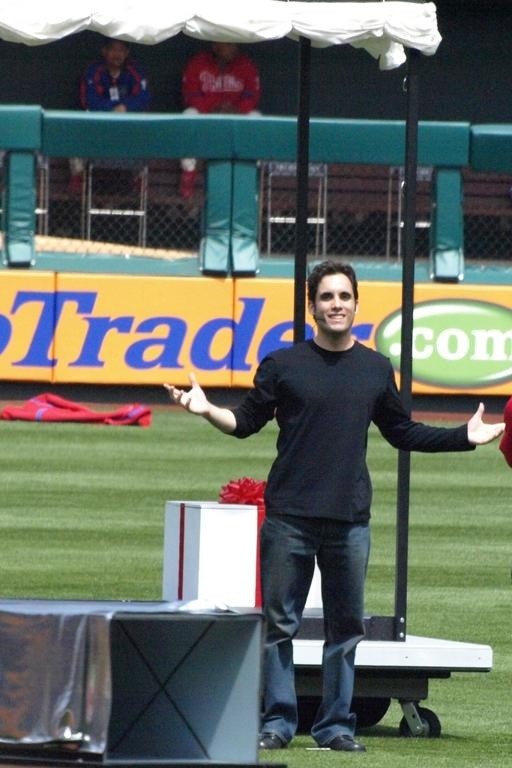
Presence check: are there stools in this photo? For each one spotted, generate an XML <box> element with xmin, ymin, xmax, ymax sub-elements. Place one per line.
<box><xmin>386</xmin><ymin>165</ymin><xmax>433</xmax><ymax>265</ymax></box>
<box><xmin>258</xmin><ymin>162</ymin><xmax>329</xmax><ymax>257</ymax></box>
<box><xmin>0</xmin><ymin>151</ymin><xmax>49</xmax><ymax>236</ymax></box>
<box><xmin>78</xmin><ymin>160</ymin><xmax>148</xmax><ymax>246</ymax></box>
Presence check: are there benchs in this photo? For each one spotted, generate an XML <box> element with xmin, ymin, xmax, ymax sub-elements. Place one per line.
<box><xmin>48</xmin><ymin>156</ymin><xmax>512</xmax><ymax>220</ymax></box>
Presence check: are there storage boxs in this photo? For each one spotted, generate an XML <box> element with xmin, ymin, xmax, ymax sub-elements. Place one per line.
<box><xmin>163</xmin><ymin>501</ymin><xmax>321</xmax><ymax>608</ymax></box>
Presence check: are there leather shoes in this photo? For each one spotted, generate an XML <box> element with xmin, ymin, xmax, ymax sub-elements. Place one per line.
<box><xmin>255</xmin><ymin>726</ymin><xmax>286</xmax><ymax>750</ymax></box>
<box><xmin>318</xmin><ymin>731</ymin><xmax>368</xmax><ymax>752</ymax></box>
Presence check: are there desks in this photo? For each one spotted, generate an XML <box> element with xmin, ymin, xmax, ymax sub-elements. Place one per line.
<box><xmin>0</xmin><ymin>598</ymin><xmax>286</xmax><ymax>768</ymax></box>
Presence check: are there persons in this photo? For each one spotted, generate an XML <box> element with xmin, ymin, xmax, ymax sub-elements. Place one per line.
<box><xmin>61</xmin><ymin>36</ymin><xmax>157</xmax><ymax>201</ymax></box>
<box><xmin>163</xmin><ymin>39</ymin><xmax>267</xmax><ymax>205</ymax></box>
<box><xmin>163</xmin><ymin>259</ymin><xmax>509</xmax><ymax>754</ymax></box>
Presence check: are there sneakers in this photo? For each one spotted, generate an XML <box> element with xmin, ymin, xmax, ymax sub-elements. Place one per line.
<box><xmin>180</xmin><ymin>169</ymin><xmax>195</xmax><ymax>199</ymax></box>
<box><xmin>67</xmin><ymin>176</ymin><xmax>80</xmax><ymax>194</ymax></box>
